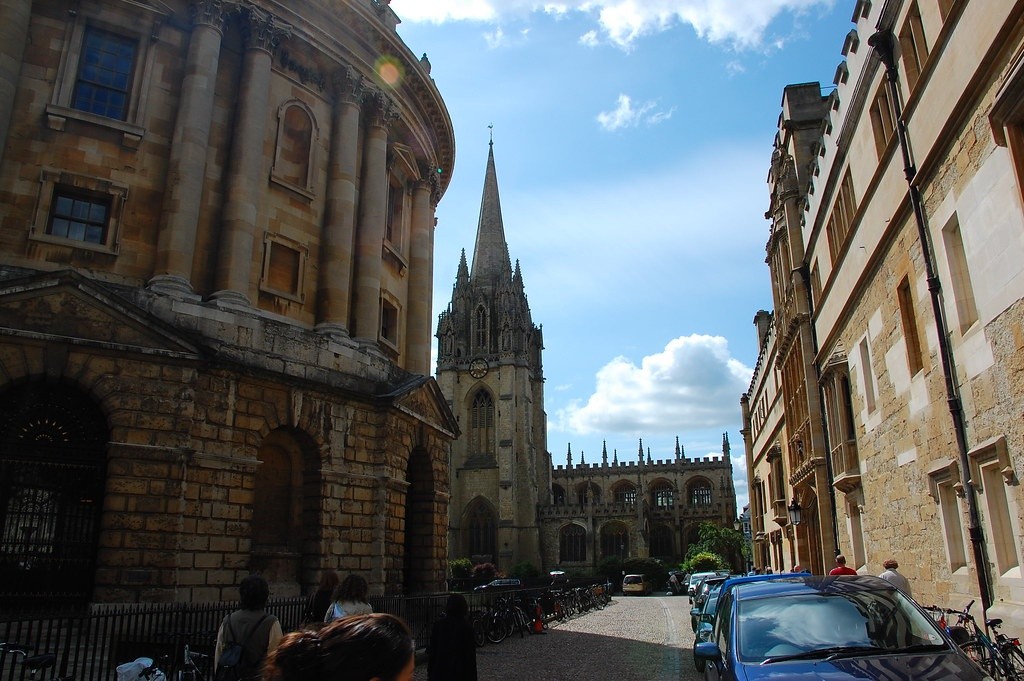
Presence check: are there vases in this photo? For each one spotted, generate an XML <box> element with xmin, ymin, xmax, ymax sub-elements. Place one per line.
<box><xmin>479</xmin><ymin>574</ymin><xmax>492</xmax><ymax>583</ymax></box>
<box><xmin>452</xmin><ymin>567</ymin><xmax>468</xmax><ymax>578</ymax></box>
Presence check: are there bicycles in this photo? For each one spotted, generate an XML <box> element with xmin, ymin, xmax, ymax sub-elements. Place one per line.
<box><xmin>472</xmin><ymin>577</ymin><xmax>612</xmax><ymax>647</ymax></box>
<box><xmin>153</xmin><ymin>630</ymin><xmax>217</xmax><ymax>681</ymax></box>
<box><xmin>921</xmin><ymin>600</ymin><xmax>1024</xmax><ymax>681</ymax></box>
<box><xmin>0</xmin><ymin>642</ymin><xmax>75</xmax><ymax>681</ymax></box>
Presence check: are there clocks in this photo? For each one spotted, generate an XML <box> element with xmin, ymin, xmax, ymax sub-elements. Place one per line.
<box><xmin>470</xmin><ymin>357</ymin><xmax>488</xmax><ymax>379</ymax></box>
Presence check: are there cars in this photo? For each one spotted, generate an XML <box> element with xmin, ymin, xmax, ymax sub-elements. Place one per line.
<box><xmin>474</xmin><ymin>578</ymin><xmax>522</xmax><ymax>592</ymax></box>
<box><xmin>688</xmin><ymin>570</ymin><xmax>995</xmax><ymax>681</ymax></box>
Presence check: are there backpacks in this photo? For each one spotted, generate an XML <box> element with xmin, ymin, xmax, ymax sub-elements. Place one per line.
<box><xmin>215</xmin><ymin>612</ymin><xmax>270</xmax><ymax>681</ymax></box>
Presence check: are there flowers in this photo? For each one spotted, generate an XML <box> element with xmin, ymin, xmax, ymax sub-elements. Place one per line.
<box><xmin>474</xmin><ymin>563</ymin><xmax>497</xmax><ymax>575</ymax></box>
<box><xmin>450</xmin><ymin>559</ymin><xmax>474</xmax><ymax>568</ymax></box>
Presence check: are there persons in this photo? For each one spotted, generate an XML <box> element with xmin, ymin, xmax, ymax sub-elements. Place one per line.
<box><xmin>669</xmin><ymin>571</ymin><xmax>691</xmax><ymax>596</ymax></box>
<box><xmin>741</xmin><ymin>565</ymin><xmax>773</xmax><ymax>578</ymax></box>
<box><xmin>427</xmin><ymin>593</ymin><xmax>478</xmax><ymax>681</ymax></box>
<box><xmin>879</xmin><ymin>560</ymin><xmax>912</xmax><ymax>599</ymax></box>
<box><xmin>264</xmin><ymin>567</ymin><xmax>414</xmax><ymax>681</ymax></box>
<box><xmin>829</xmin><ymin>555</ymin><xmax>857</xmax><ymax>576</ymax></box>
<box><xmin>214</xmin><ymin>576</ymin><xmax>284</xmax><ymax>681</ymax></box>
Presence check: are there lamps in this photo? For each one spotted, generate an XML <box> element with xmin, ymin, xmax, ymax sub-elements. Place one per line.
<box><xmin>733</xmin><ymin>518</ymin><xmax>751</xmax><ymax>533</ymax></box>
<box><xmin>788</xmin><ymin>490</ymin><xmax>817</xmax><ymax>526</ymax></box>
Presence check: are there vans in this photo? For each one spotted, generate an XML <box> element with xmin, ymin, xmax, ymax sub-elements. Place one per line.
<box><xmin>622</xmin><ymin>574</ymin><xmax>653</xmax><ymax>595</ymax></box>
